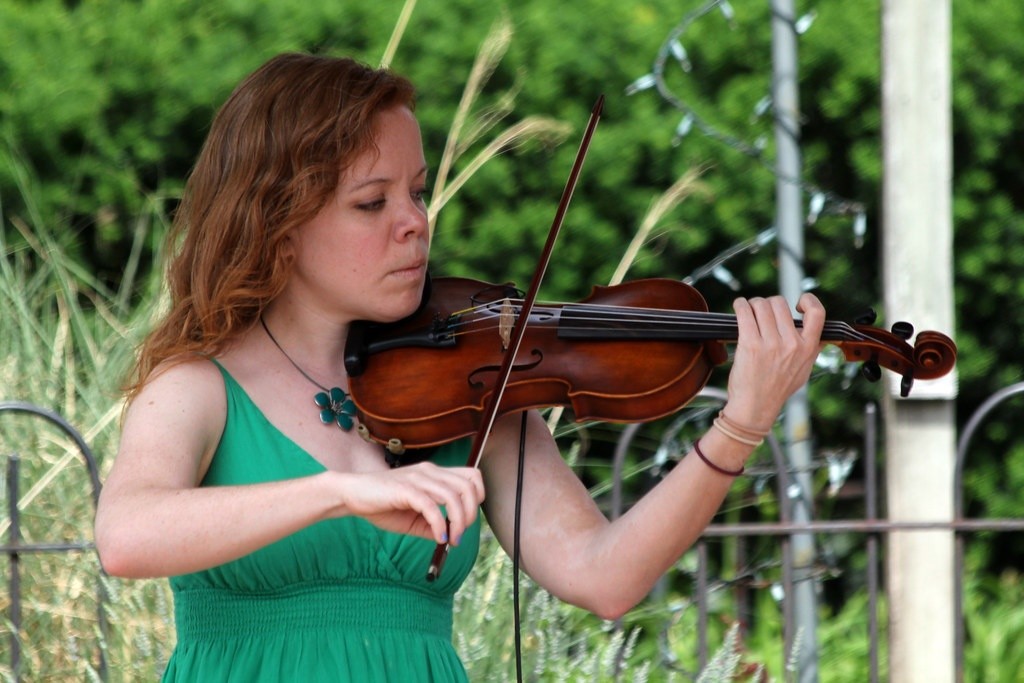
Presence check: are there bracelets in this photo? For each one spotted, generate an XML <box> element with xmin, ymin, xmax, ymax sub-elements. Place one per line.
<box><xmin>694</xmin><ymin>437</ymin><xmax>744</xmax><ymax>475</ymax></box>
<box><xmin>714</xmin><ymin>419</ymin><xmax>764</xmax><ymax>446</ymax></box>
<box><xmin>719</xmin><ymin>410</ymin><xmax>772</xmax><ymax>437</ymax></box>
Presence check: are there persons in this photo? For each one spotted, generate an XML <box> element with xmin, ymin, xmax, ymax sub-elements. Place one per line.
<box><xmin>95</xmin><ymin>52</ymin><xmax>827</xmax><ymax>683</ymax></box>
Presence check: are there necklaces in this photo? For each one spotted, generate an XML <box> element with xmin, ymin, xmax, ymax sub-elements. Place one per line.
<box><xmin>260</xmin><ymin>315</ymin><xmax>358</xmax><ymax>431</ymax></box>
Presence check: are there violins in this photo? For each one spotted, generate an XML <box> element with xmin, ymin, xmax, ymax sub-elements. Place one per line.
<box><xmin>342</xmin><ymin>273</ymin><xmax>960</xmax><ymax>456</ymax></box>
